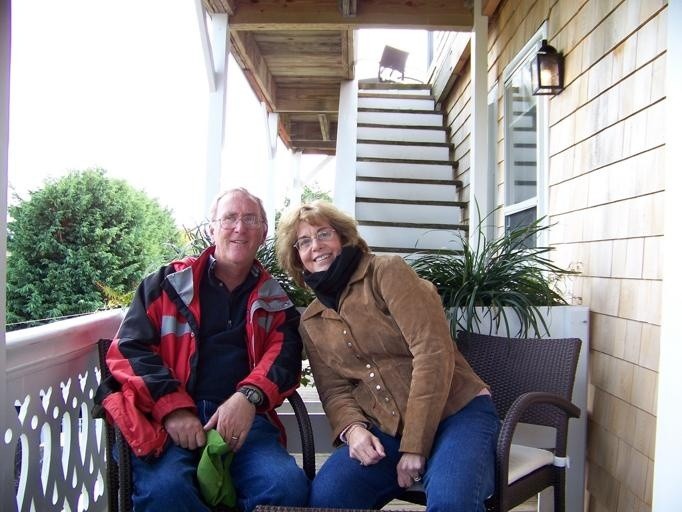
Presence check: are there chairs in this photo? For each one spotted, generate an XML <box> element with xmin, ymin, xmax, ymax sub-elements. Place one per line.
<box><xmin>95</xmin><ymin>335</ymin><xmax>318</xmax><ymax>511</ymax></box>
<box><xmin>387</xmin><ymin>327</ymin><xmax>584</xmax><ymax>511</ymax></box>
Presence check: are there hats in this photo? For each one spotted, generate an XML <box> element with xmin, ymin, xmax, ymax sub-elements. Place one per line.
<box><xmin>196</xmin><ymin>429</ymin><xmax>237</xmax><ymax>507</ymax></box>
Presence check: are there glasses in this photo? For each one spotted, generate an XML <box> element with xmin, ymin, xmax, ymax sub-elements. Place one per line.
<box><xmin>214</xmin><ymin>214</ymin><xmax>263</xmax><ymax>229</ymax></box>
<box><xmin>293</xmin><ymin>229</ymin><xmax>337</xmax><ymax>250</ymax></box>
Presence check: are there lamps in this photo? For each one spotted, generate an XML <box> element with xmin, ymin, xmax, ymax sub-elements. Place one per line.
<box><xmin>529</xmin><ymin>37</ymin><xmax>565</xmax><ymax>97</ymax></box>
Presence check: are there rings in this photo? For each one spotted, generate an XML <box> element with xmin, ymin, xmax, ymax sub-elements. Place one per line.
<box><xmin>232</xmin><ymin>435</ymin><xmax>239</xmax><ymax>440</ymax></box>
<box><xmin>412</xmin><ymin>473</ymin><xmax>421</xmax><ymax>482</ymax></box>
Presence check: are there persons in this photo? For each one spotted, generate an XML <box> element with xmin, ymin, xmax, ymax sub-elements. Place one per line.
<box><xmin>99</xmin><ymin>182</ymin><xmax>311</xmax><ymax>510</ymax></box>
<box><xmin>269</xmin><ymin>191</ymin><xmax>505</xmax><ymax>512</ymax></box>
